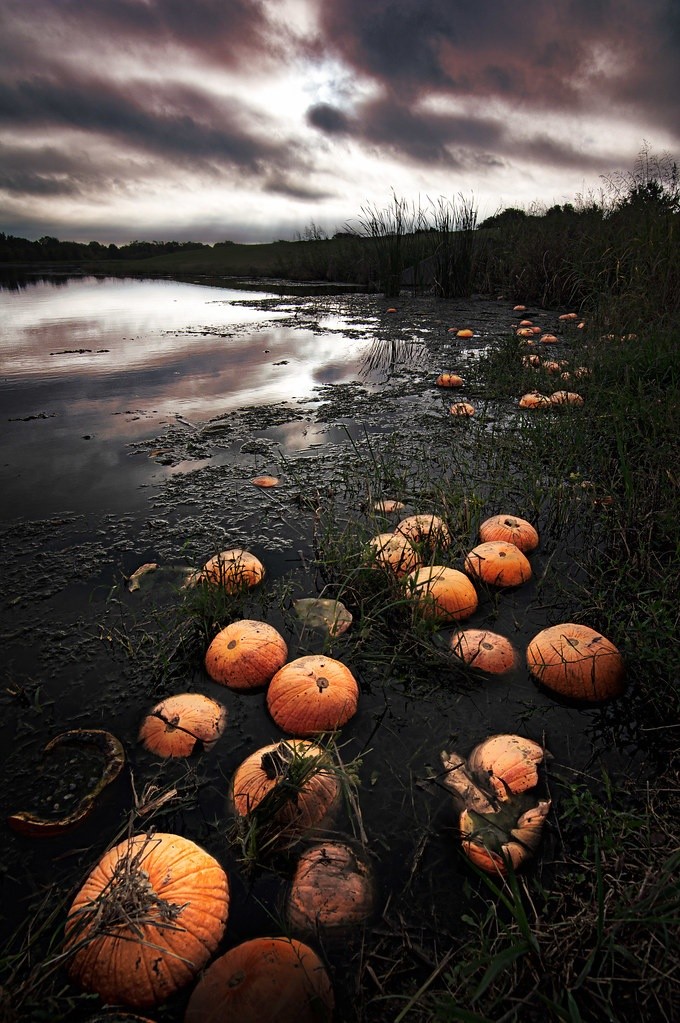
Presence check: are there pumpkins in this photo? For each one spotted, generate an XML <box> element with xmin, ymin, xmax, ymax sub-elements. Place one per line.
<box><xmin>65</xmin><ymin>498</ymin><xmax>617</xmax><ymax>1022</ymax></box>
<box><xmin>385</xmin><ymin>303</ymin><xmax>637</xmax><ymax>416</ymax></box>
<box><xmin>252</xmin><ymin>476</ymin><xmax>278</xmax><ymax>487</ymax></box>
